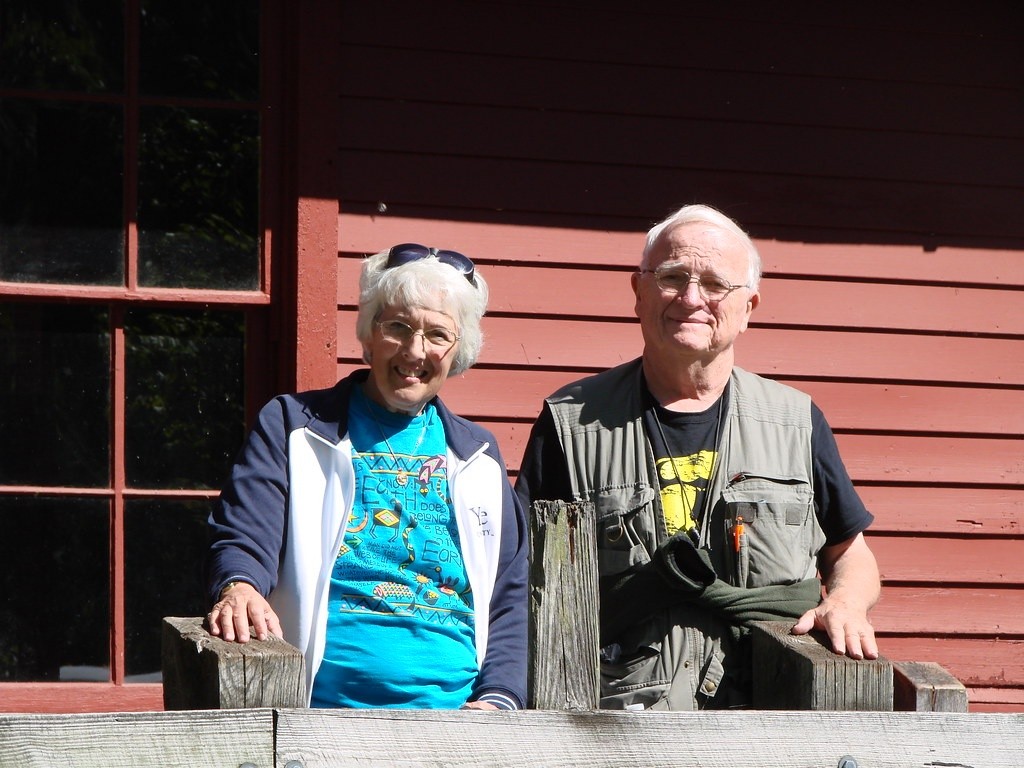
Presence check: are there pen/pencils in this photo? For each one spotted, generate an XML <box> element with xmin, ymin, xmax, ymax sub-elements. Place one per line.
<box><xmin>734</xmin><ymin>516</ymin><xmax>743</xmax><ymax>587</ymax></box>
<box><xmin>724</xmin><ymin>518</ymin><xmax>736</xmax><ymax>587</ymax></box>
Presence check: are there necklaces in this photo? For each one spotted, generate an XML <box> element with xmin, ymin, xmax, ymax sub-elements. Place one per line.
<box><xmin>643</xmin><ymin>377</ymin><xmax>723</xmax><ymax>541</ymax></box>
<box><xmin>362</xmin><ymin>388</ymin><xmax>425</xmax><ymax>484</ymax></box>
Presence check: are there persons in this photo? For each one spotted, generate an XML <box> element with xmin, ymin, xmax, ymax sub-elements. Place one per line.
<box><xmin>206</xmin><ymin>242</ymin><xmax>530</xmax><ymax>710</ymax></box>
<box><xmin>514</xmin><ymin>204</ymin><xmax>882</xmax><ymax>711</ymax></box>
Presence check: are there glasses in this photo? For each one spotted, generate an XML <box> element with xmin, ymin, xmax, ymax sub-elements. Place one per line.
<box><xmin>643</xmin><ymin>268</ymin><xmax>750</xmax><ymax>302</ymax></box>
<box><xmin>386</xmin><ymin>243</ymin><xmax>474</xmax><ymax>284</ymax></box>
<box><xmin>374</xmin><ymin>321</ymin><xmax>461</xmax><ymax>351</ymax></box>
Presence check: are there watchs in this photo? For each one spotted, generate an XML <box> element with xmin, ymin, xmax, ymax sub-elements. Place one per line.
<box><xmin>218</xmin><ymin>580</ymin><xmax>236</xmax><ymax>592</ymax></box>
